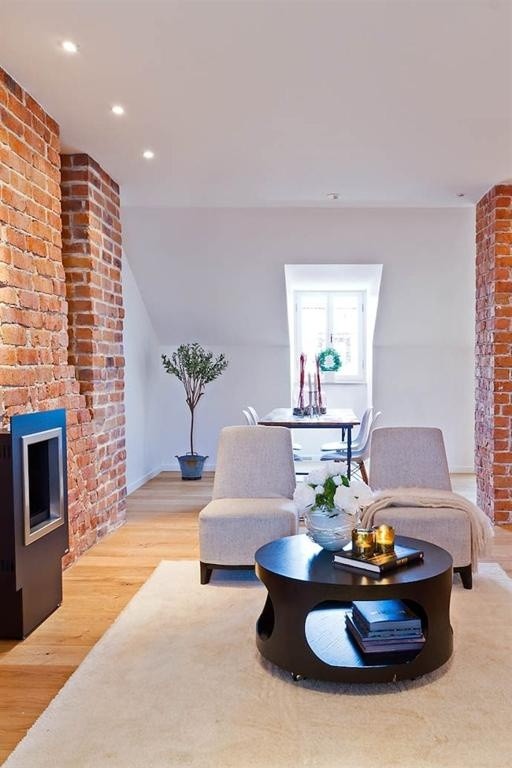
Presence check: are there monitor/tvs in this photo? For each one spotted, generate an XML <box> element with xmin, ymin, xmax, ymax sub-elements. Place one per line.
<box><xmin>28</xmin><ymin>440</ymin><xmax>51</xmax><ymax>528</ymax></box>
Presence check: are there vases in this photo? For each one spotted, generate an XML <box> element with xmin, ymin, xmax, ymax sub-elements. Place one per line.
<box><xmin>304</xmin><ymin>505</ymin><xmax>358</xmax><ymax>551</ymax></box>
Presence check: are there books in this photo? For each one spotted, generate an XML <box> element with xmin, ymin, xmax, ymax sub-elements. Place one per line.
<box><xmin>343</xmin><ymin>599</ymin><xmax>427</xmax><ymax>654</ymax></box>
<box><xmin>329</xmin><ymin>541</ymin><xmax>423</xmax><ymax>574</ymax></box>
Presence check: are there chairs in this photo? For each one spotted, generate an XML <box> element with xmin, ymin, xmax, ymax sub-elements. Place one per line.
<box><xmin>242</xmin><ymin>406</ymin><xmax>301</xmax><ymax>459</ymax></box>
<box><xmin>320</xmin><ymin>407</ymin><xmax>382</xmax><ymax>481</ymax></box>
<box><xmin>198</xmin><ymin>426</ymin><xmax>299</xmax><ymax>584</ymax></box>
<box><xmin>369</xmin><ymin>428</ymin><xmax>473</xmax><ymax>588</ymax></box>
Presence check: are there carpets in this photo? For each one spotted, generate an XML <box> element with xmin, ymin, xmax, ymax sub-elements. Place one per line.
<box><xmin>0</xmin><ymin>556</ymin><xmax>511</xmax><ymax>768</ymax></box>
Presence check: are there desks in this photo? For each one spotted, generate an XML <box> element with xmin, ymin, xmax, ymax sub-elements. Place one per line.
<box><xmin>258</xmin><ymin>407</ymin><xmax>360</xmax><ymax>480</ymax></box>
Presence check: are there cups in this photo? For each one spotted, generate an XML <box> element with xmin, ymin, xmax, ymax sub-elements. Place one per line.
<box><xmin>351</xmin><ymin>529</ymin><xmax>374</xmax><ymax>556</ymax></box>
<box><xmin>372</xmin><ymin>523</ymin><xmax>395</xmax><ymax>552</ymax></box>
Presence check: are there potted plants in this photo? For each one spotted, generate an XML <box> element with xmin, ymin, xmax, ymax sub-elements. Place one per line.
<box><xmin>160</xmin><ymin>341</ymin><xmax>231</xmax><ymax>480</ymax></box>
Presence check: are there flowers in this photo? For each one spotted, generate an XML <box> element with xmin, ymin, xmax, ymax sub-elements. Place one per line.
<box><xmin>293</xmin><ymin>460</ymin><xmax>375</xmax><ymax>513</ymax></box>
